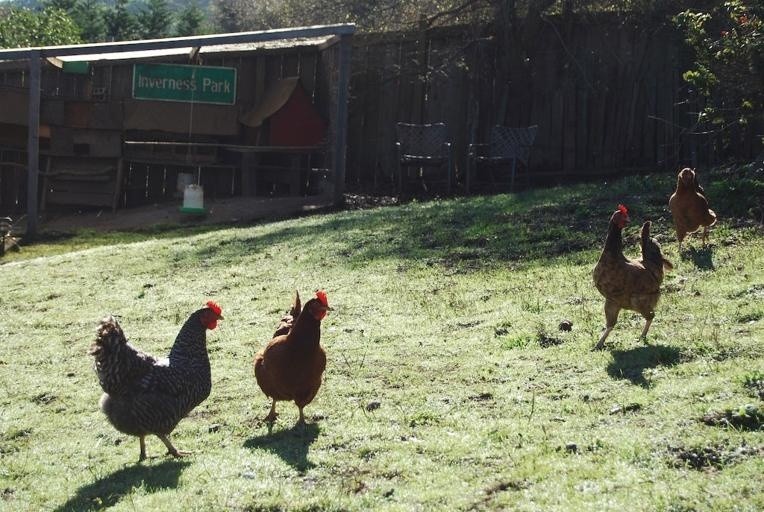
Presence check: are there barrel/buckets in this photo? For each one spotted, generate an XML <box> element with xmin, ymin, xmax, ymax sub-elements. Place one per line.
<box><xmin>183</xmin><ymin>185</ymin><xmax>204</xmax><ymax>208</ymax></box>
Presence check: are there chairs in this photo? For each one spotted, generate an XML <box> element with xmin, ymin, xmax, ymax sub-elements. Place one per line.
<box><xmin>120</xmin><ymin>127</ymin><xmax>331</xmax><ymax>201</ymax></box>
<box><xmin>394</xmin><ymin>120</ymin><xmax>539</xmax><ymax>197</ymax></box>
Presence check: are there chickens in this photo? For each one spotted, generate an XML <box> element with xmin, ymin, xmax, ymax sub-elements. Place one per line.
<box><xmin>589</xmin><ymin>202</ymin><xmax>674</xmax><ymax>352</ymax></box>
<box><xmin>86</xmin><ymin>301</ymin><xmax>225</xmax><ymax>463</ymax></box>
<box><xmin>668</xmin><ymin>167</ymin><xmax>718</xmax><ymax>254</ymax></box>
<box><xmin>254</xmin><ymin>289</ymin><xmax>333</xmax><ymax>427</ymax></box>
